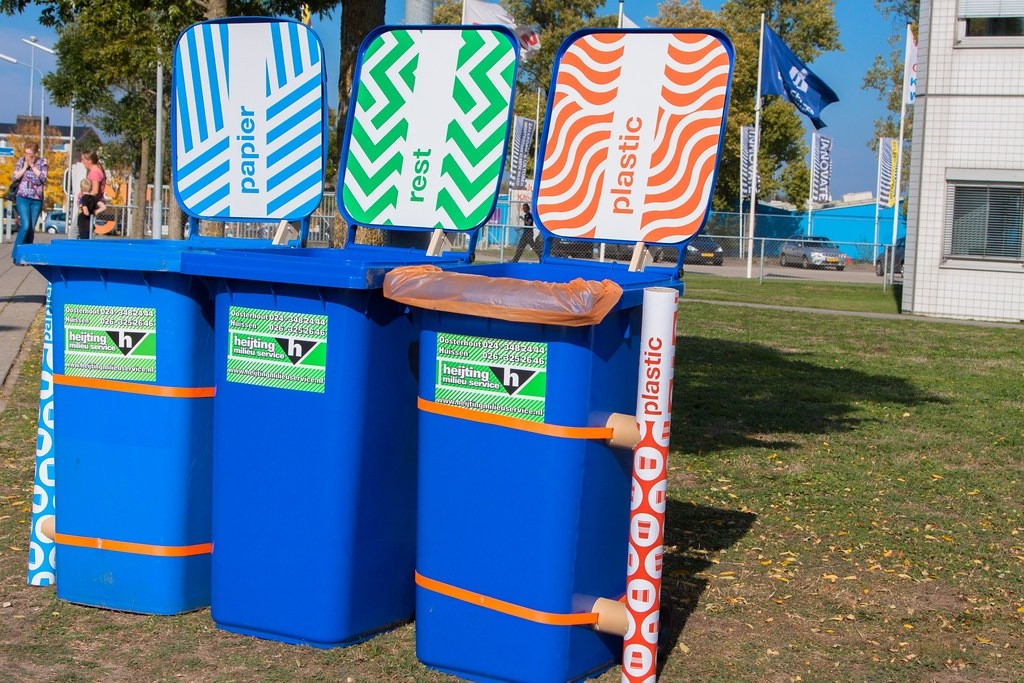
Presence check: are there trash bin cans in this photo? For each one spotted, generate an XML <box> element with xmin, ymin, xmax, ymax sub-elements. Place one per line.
<box><xmin>10</xmin><ymin>21</ymin><xmax>327</xmax><ymax>617</ymax></box>
<box><xmin>379</xmin><ymin>26</ymin><xmax>738</xmax><ymax>683</ymax></box>
<box><xmin>179</xmin><ymin>24</ymin><xmax>517</xmax><ymax>650</ymax></box>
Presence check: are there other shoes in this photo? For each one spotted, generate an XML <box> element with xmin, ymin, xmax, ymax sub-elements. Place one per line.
<box><xmin>13</xmin><ymin>259</ymin><xmax>29</xmax><ymax>266</ymax></box>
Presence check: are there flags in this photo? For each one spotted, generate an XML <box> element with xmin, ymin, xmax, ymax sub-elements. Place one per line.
<box><xmin>621</xmin><ymin>15</ymin><xmax>641</xmax><ymax>32</ymax></box>
<box><xmin>463</xmin><ymin>0</ymin><xmax>542</xmax><ymax>63</ymax></box>
<box><xmin>813</xmin><ymin>133</ymin><xmax>833</xmax><ymax>203</ymax></box>
<box><xmin>879</xmin><ymin>138</ymin><xmax>900</xmax><ymax>209</ymax></box>
<box><xmin>741</xmin><ymin>126</ymin><xmax>761</xmax><ymax>196</ymax></box>
<box><xmin>761</xmin><ymin>23</ymin><xmax>840</xmax><ymax>130</ymax></box>
<box><xmin>904</xmin><ymin>29</ymin><xmax>918</xmax><ymax>103</ymax></box>
<box><xmin>302</xmin><ymin>4</ymin><xmax>312</xmax><ymax>27</ymax></box>
<box><xmin>510</xmin><ymin>115</ymin><xmax>536</xmax><ymax>189</ymax></box>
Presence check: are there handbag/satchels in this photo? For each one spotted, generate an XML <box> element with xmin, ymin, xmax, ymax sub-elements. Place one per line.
<box><xmin>5</xmin><ymin>180</ymin><xmax>20</xmax><ymax>201</ymax></box>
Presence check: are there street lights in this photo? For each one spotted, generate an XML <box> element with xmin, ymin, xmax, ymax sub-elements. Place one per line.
<box><xmin>0</xmin><ymin>53</ymin><xmax>45</xmax><ymax>162</ymax></box>
<box><xmin>21</xmin><ymin>38</ymin><xmax>76</xmax><ymax>235</ymax></box>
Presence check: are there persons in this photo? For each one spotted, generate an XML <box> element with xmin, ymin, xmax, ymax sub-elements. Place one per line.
<box><xmin>11</xmin><ymin>142</ymin><xmax>49</xmax><ymax>267</ymax></box>
<box><xmin>77</xmin><ymin>178</ymin><xmax>107</xmax><ymax>239</ymax></box>
<box><xmin>504</xmin><ymin>203</ymin><xmax>543</xmax><ymax>263</ymax></box>
<box><xmin>68</xmin><ymin>148</ymin><xmax>106</xmax><ymax>239</ymax></box>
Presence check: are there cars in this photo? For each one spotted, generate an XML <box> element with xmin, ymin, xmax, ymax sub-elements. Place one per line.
<box><xmin>536</xmin><ymin>234</ymin><xmax>663</xmax><ymax>263</ymax></box>
<box><xmin>778</xmin><ymin>235</ymin><xmax>846</xmax><ymax>270</ymax></box>
<box><xmin>245</xmin><ymin>223</ymin><xmax>329</xmax><ymax>243</ymax></box>
<box><xmin>660</xmin><ymin>230</ymin><xmax>723</xmax><ymax>266</ymax></box>
<box><xmin>3</xmin><ymin>208</ymin><xmax>20</xmax><ymax>235</ymax></box>
<box><xmin>876</xmin><ymin>236</ymin><xmax>906</xmax><ymax>279</ymax></box>
<box><xmin>45</xmin><ymin>213</ymin><xmax>71</xmax><ymax>234</ymax></box>
